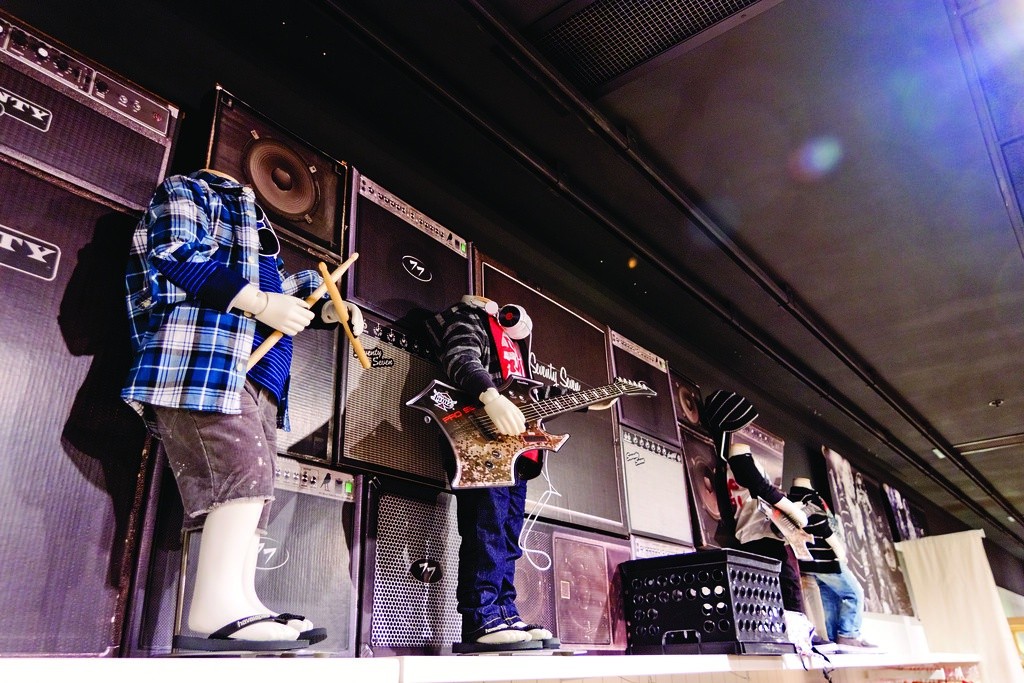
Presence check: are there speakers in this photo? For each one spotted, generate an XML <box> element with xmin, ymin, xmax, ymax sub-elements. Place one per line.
<box><xmin>0</xmin><ymin>11</ymin><xmax>789</xmax><ymax>660</ymax></box>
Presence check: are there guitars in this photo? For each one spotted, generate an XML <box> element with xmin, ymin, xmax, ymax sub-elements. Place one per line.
<box><xmin>756</xmin><ymin>495</ymin><xmax>825</xmax><ymax>560</ymax></box>
<box><xmin>402</xmin><ymin>375</ymin><xmax>659</xmax><ymax>489</ymax></box>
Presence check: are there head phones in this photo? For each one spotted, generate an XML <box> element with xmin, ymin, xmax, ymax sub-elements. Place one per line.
<box><xmin>462</xmin><ymin>294</ymin><xmax>533</xmax><ymax>340</ymax></box>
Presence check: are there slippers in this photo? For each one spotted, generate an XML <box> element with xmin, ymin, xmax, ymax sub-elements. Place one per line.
<box><xmin>451</xmin><ymin>627</ymin><xmax>544</xmax><ymax>654</ymax></box>
<box><xmin>520</xmin><ymin>624</ymin><xmax>561</xmax><ymax>649</ymax></box>
<box><xmin>276</xmin><ymin>612</ymin><xmax>328</xmax><ymax>645</ymax></box>
<box><xmin>172</xmin><ymin>614</ymin><xmax>310</xmax><ymax>650</ymax></box>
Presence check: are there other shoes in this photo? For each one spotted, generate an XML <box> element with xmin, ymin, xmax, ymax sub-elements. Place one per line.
<box><xmin>837</xmin><ymin>637</ymin><xmax>886</xmax><ymax>654</ymax></box>
<box><xmin>809</xmin><ymin>634</ymin><xmax>838</xmax><ymax>653</ymax></box>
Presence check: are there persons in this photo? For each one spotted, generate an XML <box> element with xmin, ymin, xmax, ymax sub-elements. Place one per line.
<box><xmin>831</xmin><ymin>459</ymin><xmax>914</xmax><ymax>617</ymax></box>
<box><xmin>702</xmin><ymin>389</ymin><xmax>841</xmax><ymax>653</ymax></box>
<box><xmin>785</xmin><ymin>475</ymin><xmax>882</xmax><ymax>643</ymax></box>
<box><xmin>427</xmin><ymin>295</ymin><xmax>622</xmax><ymax>649</ymax></box>
<box><xmin>893</xmin><ymin>489</ymin><xmax>912</xmax><ymax>538</ymax></box>
<box><xmin>124</xmin><ymin>167</ymin><xmax>367</xmax><ymax>650</ymax></box>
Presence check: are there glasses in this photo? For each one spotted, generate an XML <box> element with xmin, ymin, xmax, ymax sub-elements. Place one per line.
<box><xmin>246</xmin><ymin>201</ymin><xmax>281</xmax><ymax>260</ymax></box>
